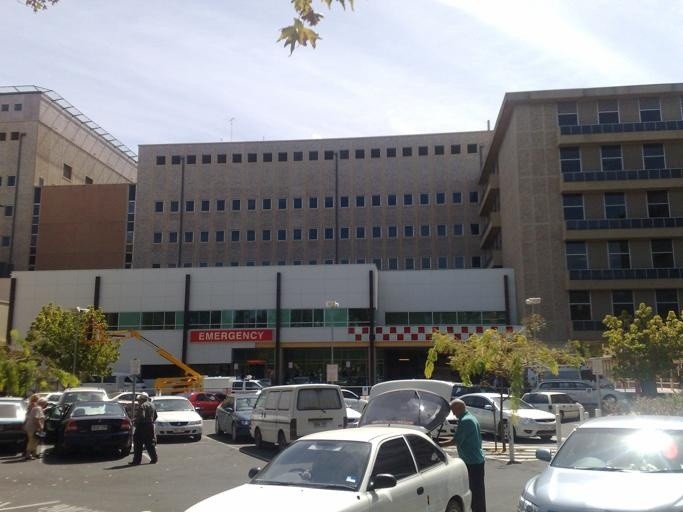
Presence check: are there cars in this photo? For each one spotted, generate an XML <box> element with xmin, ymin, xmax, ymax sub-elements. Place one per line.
<box><xmin>177</xmin><ymin>378</ymin><xmax>479</xmax><ymax>510</ymax></box>
<box><xmin>520</xmin><ymin>413</ymin><xmax>683</xmax><ymax>512</ymax></box>
<box><xmin>428</xmin><ymin>379</ymin><xmax>628</xmax><ymax>442</ymax></box>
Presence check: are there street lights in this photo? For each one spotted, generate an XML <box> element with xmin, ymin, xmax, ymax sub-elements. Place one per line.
<box><xmin>72</xmin><ymin>307</ymin><xmax>90</xmax><ymax>375</ymax></box>
<box><xmin>323</xmin><ymin>298</ymin><xmax>340</xmax><ymax>384</ymax></box>
<box><xmin>525</xmin><ymin>295</ymin><xmax>542</xmax><ymax>392</ymax></box>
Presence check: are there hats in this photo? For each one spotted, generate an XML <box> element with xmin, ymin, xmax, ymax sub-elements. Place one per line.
<box><xmin>136</xmin><ymin>391</ymin><xmax>149</xmax><ymax>400</ymax></box>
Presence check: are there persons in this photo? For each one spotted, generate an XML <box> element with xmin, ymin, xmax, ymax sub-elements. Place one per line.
<box><xmin>437</xmin><ymin>399</ymin><xmax>485</xmax><ymax>512</ymax></box>
<box><xmin>128</xmin><ymin>392</ymin><xmax>158</xmax><ymax>465</ymax></box>
<box><xmin>22</xmin><ymin>398</ymin><xmax>47</xmax><ymax>460</ymax></box>
<box><xmin>27</xmin><ymin>394</ymin><xmax>38</xmax><ymax>413</ymax></box>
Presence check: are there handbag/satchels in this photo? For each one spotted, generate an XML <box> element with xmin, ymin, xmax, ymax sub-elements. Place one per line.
<box><xmin>33</xmin><ymin>431</ymin><xmax>50</xmax><ymax>442</ymax></box>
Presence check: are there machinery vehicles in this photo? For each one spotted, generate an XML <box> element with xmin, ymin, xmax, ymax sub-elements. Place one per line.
<box><xmin>82</xmin><ymin>321</ymin><xmax>204</xmax><ymax>397</ymax></box>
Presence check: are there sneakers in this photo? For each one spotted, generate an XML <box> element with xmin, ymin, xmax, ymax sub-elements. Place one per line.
<box><xmin>128</xmin><ymin>462</ymin><xmax>142</xmax><ymax>466</ymax></box>
<box><xmin>22</xmin><ymin>454</ymin><xmax>41</xmax><ymax>461</ymax></box>
<box><xmin>148</xmin><ymin>456</ymin><xmax>158</xmax><ymax>465</ymax></box>
